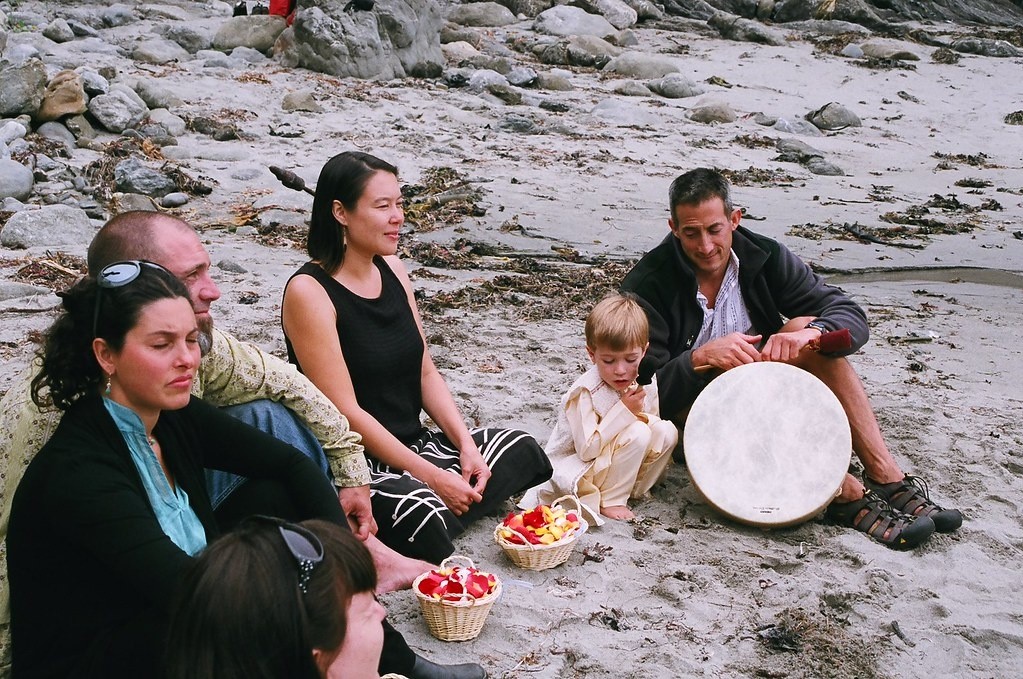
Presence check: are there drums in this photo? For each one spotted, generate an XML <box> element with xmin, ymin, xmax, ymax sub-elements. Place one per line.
<box><xmin>682</xmin><ymin>360</ymin><xmax>854</xmax><ymax>530</ymax></box>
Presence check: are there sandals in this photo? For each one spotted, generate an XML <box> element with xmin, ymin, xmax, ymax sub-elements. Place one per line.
<box><xmin>861</xmin><ymin>468</ymin><xmax>962</xmax><ymax>534</ymax></box>
<box><xmin>821</xmin><ymin>487</ymin><xmax>935</xmax><ymax>552</ymax></box>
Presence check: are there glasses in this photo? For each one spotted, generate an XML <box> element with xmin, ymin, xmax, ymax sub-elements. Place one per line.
<box><xmin>239</xmin><ymin>515</ymin><xmax>324</xmax><ymax>601</ymax></box>
<box><xmin>92</xmin><ymin>260</ymin><xmax>175</xmax><ymax>341</ymax></box>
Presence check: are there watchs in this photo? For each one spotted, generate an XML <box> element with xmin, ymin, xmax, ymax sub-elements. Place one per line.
<box><xmin>802</xmin><ymin>322</ymin><xmax>828</xmax><ymax>336</ymax></box>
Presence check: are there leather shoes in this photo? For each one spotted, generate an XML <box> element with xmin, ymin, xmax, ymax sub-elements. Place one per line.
<box><xmin>410</xmin><ymin>653</ymin><xmax>488</xmax><ymax>679</ymax></box>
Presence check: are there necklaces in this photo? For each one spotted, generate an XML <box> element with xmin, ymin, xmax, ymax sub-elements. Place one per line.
<box><xmin>144</xmin><ymin>434</ymin><xmax>157</xmax><ymax>447</ymax></box>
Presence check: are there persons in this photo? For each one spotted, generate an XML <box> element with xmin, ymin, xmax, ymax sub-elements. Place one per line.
<box><xmin>516</xmin><ymin>295</ymin><xmax>679</xmax><ymax>529</ymax></box>
<box><xmin>0</xmin><ymin>151</ymin><xmax>554</xmax><ymax>679</ymax></box>
<box><xmin>619</xmin><ymin>167</ymin><xmax>963</xmax><ymax>553</ymax></box>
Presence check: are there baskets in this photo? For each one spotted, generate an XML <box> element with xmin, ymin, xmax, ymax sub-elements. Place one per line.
<box><xmin>412</xmin><ymin>556</ymin><xmax>504</xmax><ymax>643</ymax></box>
<box><xmin>493</xmin><ymin>495</ymin><xmax>589</xmax><ymax>571</ymax></box>
